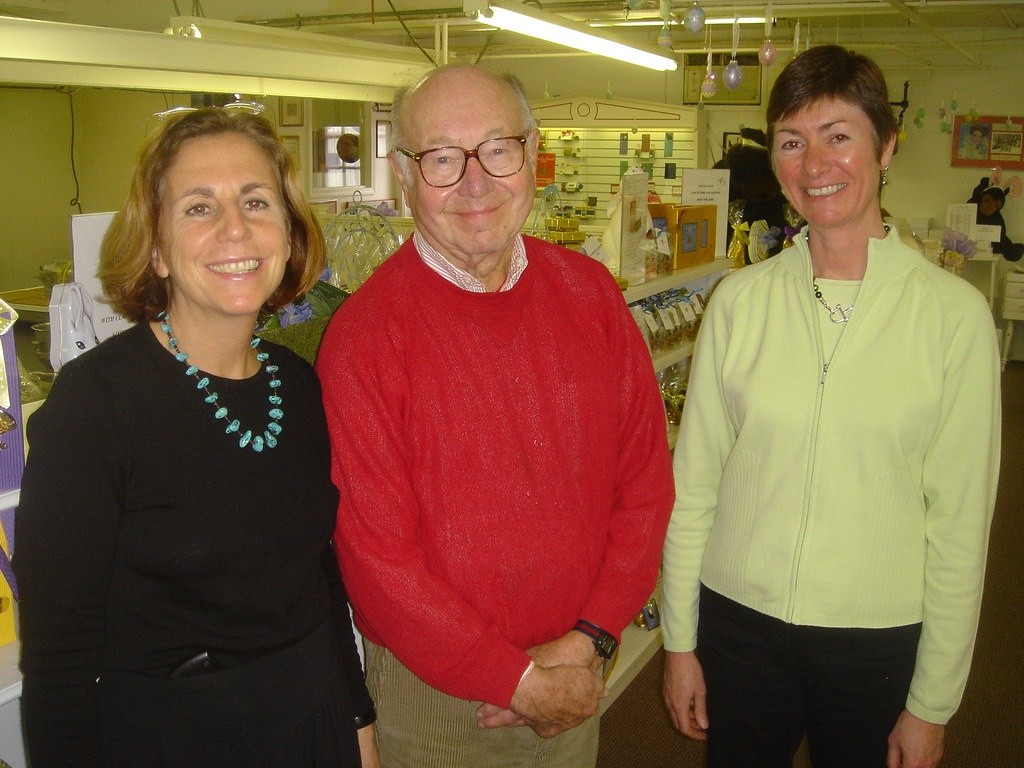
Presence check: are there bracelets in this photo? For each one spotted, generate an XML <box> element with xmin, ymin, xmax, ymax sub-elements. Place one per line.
<box><xmin>354</xmin><ymin>710</ymin><xmax>377</xmax><ymax>730</ymax></box>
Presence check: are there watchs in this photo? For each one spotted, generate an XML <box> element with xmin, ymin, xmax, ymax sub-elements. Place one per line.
<box><xmin>574</xmin><ymin>620</ymin><xmax>618</xmax><ymax>660</ymax></box>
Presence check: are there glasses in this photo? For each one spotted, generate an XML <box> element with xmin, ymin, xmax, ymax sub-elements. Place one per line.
<box><xmin>151</xmin><ymin>101</ymin><xmax>267</xmax><ymax>122</ymax></box>
<box><xmin>398</xmin><ymin>127</ymin><xmax>530</xmax><ymax>188</ymax></box>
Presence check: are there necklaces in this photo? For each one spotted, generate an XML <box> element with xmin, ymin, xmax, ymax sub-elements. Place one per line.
<box><xmin>156</xmin><ymin>312</ymin><xmax>284</xmax><ymax>453</ymax></box>
<box><xmin>805</xmin><ymin>225</ymin><xmax>891</xmax><ymax>323</ymax></box>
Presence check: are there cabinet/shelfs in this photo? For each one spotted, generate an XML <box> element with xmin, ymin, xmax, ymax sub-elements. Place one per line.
<box><xmin>1000</xmin><ymin>270</ymin><xmax>1024</xmax><ymax>371</ymax></box>
<box><xmin>623</xmin><ymin>259</ymin><xmax>728</xmax><ymax>450</ymax></box>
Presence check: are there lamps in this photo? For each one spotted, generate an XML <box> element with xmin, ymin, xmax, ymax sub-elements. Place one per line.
<box><xmin>463</xmin><ymin>0</ymin><xmax>679</xmax><ymax>73</ymax></box>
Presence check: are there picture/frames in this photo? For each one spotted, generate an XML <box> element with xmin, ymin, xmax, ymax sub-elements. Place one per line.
<box><xmin>950</xmin><ymin>114</ymin><xmax>1024</xmax><ymax>171</ymax></box>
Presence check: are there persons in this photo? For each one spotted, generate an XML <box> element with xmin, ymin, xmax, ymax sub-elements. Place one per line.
<box><xmin>11</xmin><ymin>106</ymin><xmax>382</xmax><ymax>768</ymax></box>
<box><xmin>661</xmin><ymin>44</ymin><xmax>1002</xmax><ymax>768</ymax></box>
<box><xmin>314</xmin><ymin>63</ymin><xmax>677</xmax><ymax>768</ymax></box>
